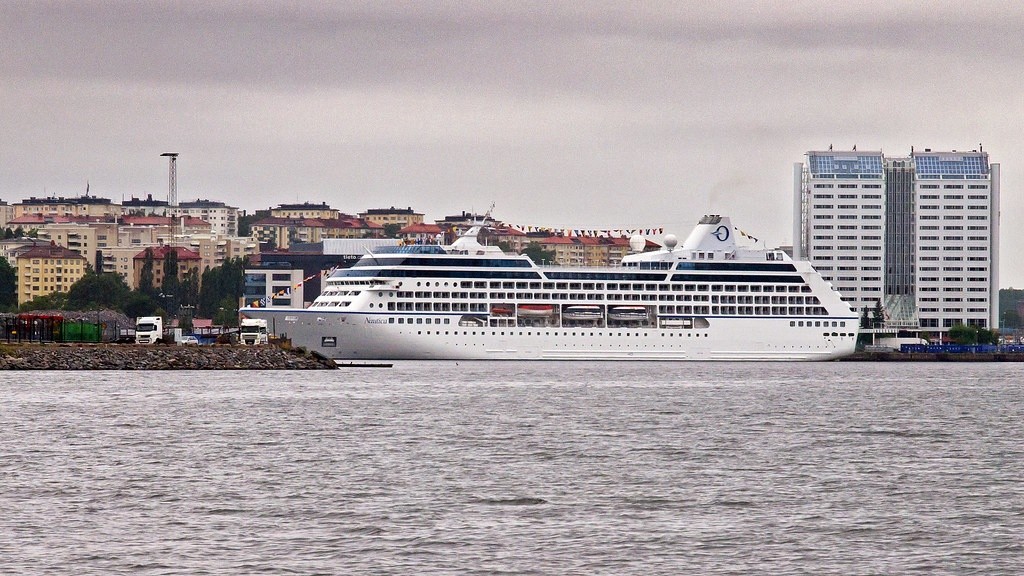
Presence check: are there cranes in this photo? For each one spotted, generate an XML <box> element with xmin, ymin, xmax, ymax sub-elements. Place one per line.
<box><xmin>159</xmin><ymin>152</ymin><xmax>180</xmax><ymax>274</ymax></box>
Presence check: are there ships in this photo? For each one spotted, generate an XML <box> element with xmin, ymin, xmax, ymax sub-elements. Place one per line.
<box><xmin>238</xmin><ymin>201</ymin><xmax>860</xmax><ymax>362</ymax></box>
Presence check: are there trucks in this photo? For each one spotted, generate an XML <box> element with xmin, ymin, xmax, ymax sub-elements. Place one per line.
<box><xmin>239</xmin><ymin>318</ymin><xmax>268</xmax><ymax>347</ymax></box>
<box><xmin>134</xmin><ymin>316</ymin><xmax>163</xmax><ymax>345</ymax></box>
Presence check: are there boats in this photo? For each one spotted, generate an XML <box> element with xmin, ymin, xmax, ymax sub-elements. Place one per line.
<box><xmin>562</xmin><ymin>305</ymin><xmax>604</xmax><ymax>321</ymax></box>
<box><xmin>608</xmin><ymin>306</ymin><xmax>647</xmax><ymax>321</ymax></box>
<box><xmin>519</xmin><ymin>304</ymin><xmax>554</xmax><ymax>318</ymax></box>
<box><xmin>491</xmin><ymin>306</ymin><xmax>514</xmax><ymax>315</ymax></box>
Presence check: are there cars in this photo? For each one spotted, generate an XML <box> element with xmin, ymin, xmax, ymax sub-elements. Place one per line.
<box><xmin>181</xmin><ymin>336</ymin><xmax>199</xmax><ymax>347</ymax></box>
